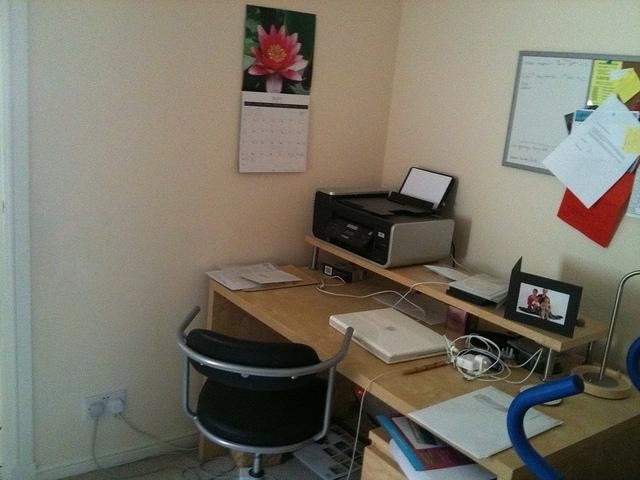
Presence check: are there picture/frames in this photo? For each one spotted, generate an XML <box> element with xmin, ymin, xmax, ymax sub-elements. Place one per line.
<box><xmin>504</xmin><ymin>271</ymin><xmax>583</xmax><ymax>338</ymax></box>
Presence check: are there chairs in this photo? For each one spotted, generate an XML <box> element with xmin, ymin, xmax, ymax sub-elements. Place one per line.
<box><xmin>176</xmin><ymin>306</ymin><xmax>354</xmax><ymax>480</ymax></box>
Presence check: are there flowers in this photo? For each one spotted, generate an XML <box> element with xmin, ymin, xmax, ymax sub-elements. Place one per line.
<box><xmin>247</xmin><ymin>24</ymin><xmax>308</xmax><ymax>94</ymax></box>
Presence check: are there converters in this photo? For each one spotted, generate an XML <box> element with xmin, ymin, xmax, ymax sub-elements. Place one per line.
<box><xmin>457</xmin><ymin>353</ymin><xmax>492</xmax><ymax>371</ymax></box>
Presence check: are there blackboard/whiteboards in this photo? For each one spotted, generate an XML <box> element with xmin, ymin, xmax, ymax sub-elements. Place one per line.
<box><xmin>502</xmin><ymin>51</ymin><xmax>640</xmax><ymax>176</ymax></box>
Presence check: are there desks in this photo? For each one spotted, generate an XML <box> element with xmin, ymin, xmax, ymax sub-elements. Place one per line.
<box><xmin>198</xmin><ymin>234</ymin><xmax>640</xmax><ymax>480</ymax></box>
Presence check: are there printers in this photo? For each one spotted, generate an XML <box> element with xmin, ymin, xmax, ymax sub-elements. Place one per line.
<box><xmin>311</xmin><ymin>166</ymin><xmax>458</xmax><ymax>270</ymax></box>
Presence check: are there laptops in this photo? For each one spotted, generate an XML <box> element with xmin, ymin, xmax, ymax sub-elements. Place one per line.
<box><xmin>329</xmin><ymin>307</ymin><xmax>456</xmax><ymax>365</ymax></box>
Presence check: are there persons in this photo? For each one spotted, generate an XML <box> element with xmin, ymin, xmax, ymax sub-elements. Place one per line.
<box><xmin>519</xmin><ymin>288</ymin><xmax>563</xmax><ymax>320</ymax></box>
